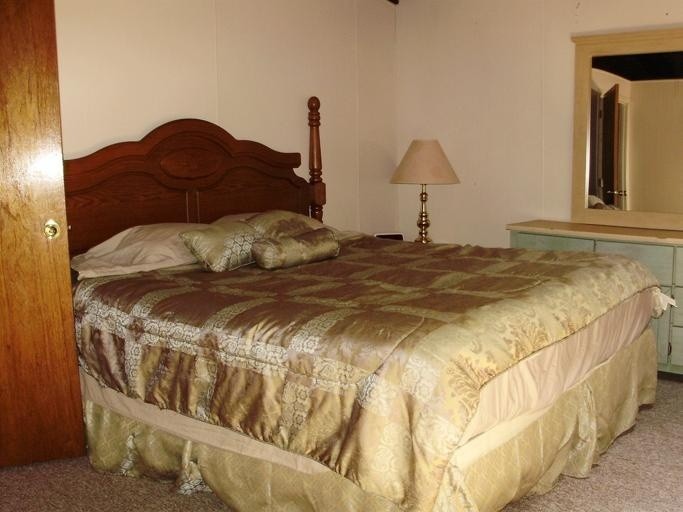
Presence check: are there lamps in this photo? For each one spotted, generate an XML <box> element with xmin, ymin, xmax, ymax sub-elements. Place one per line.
<box><xmin>389</xmin><ymin>138</ymin><xmax>460</xmax><ymax>245</ymax></box>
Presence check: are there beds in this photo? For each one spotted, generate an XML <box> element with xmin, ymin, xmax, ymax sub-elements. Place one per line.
<box><xmin>63</xmin><ymin>95</ymin><xmax>658</xmax><ymax>511</ymax></box>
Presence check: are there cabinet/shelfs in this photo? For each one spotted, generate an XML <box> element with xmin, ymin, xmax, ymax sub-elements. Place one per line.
<box><xmin>505</xmin><ymin>230</ymin><xmax>683</xmax><ymax>376</ymax></box>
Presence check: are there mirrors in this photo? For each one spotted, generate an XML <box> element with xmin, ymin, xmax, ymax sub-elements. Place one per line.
<box><xmin>569</xmin><ymin>27</ymin><xmax>683</xmax><ymax>231</ymax></box>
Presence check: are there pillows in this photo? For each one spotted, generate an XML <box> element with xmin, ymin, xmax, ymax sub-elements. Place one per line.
<box><xmin>252</xmin><ymin>227</ymin><xmax>341</xmax><ymax>270</ymax></box>
<box><xmin>178</xmin><ymin>220</ymin><xmax>263</xmax><ymax>273</ymax></box>
<box><xmin>212</xmin><ymin>211</ymin><xmax>265</xmax><ymax>223</ymax></box>
<box><xmin>122</xmin><ymin>223</ymin><xmax>209</xmax><ymax>267</ymax></box>
<box><xmin>241</xmin><ymin>210</ymin><xmax>338</xmax><ymax>241</ymax></box>
